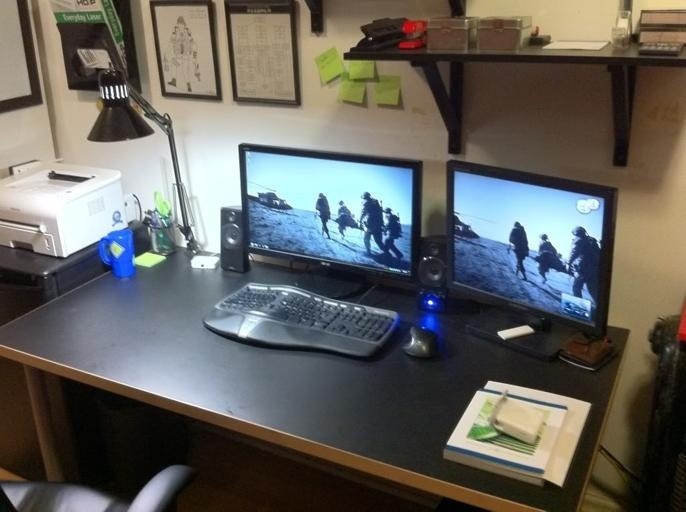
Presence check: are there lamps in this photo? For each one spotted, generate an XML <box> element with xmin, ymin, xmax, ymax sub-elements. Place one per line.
<box><xmin>80</xmin><ymin>58</ymin><xmax>208</xmax><ymax>256</ymax></box>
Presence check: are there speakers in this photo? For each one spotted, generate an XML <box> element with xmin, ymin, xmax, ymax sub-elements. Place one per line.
<box><xmin>416</xmin><ymin>235</ymin><xmax>448</xmax><ymax>314</ymax></box>
<box><xmin>220</xmin><ymin>206</ymin><xmax>249</xmax><ymax>273</ymax></box>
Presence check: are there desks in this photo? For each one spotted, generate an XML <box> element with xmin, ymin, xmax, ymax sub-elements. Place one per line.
<box><xmin>0</xmin><ymin>241</ymin><xmax>638</xmax><ymax>512</ymax></box>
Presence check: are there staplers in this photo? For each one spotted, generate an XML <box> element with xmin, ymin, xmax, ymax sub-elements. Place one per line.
<box><xmin>398</xmin><ymin>20</ymin><xmax>428</xmax><ymax>49</ymax></box>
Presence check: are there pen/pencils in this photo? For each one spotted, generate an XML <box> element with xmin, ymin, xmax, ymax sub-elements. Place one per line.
<box><xmin>145</xmin><ymin>208</ymin><xmax>177</xmax><ymax>244</ymax></box>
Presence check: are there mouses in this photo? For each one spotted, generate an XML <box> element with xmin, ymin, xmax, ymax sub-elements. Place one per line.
<box><xmin>401</xmin><ymin>326</ymin><xmax>438</xmax><ymax>360</ymax></box>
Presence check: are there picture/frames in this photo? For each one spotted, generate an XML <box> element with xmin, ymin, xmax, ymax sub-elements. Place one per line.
<box><xmin>148</xmin><ymin>0</ymin><xmax>225</xmax><ymax>102</ymax></box>
<box><xmin>0</xmin><ymin>0</ymin><xmax>46</xmax><ymax>114</ymax></box>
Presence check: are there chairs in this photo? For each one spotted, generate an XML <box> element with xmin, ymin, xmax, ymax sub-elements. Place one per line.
<box><xmin>0</xmin><ymin>457</ymin><xmax>198</xmax><ymax>512</ymax></box>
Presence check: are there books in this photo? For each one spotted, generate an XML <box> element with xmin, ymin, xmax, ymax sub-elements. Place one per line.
<box><xmin>442</xmin><ymin>387</ymin><xmax>569</xmax><ymax>486</ymax></box>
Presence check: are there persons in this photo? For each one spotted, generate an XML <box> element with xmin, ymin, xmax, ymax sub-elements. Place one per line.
<box><xmin>315</xmin><ymin>192</ymin><xmax>404</xmax><ymax>260</ymax></box>
<box><xmin>510</xmin><ymin>222</ymin><xmax>603</xmax><ymax>302</ymax></box>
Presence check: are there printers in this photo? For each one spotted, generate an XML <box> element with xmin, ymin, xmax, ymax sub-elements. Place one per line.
<box><xmin>0</xmin><ymin>162</ymin><xmax>128</xmax><ymax>258</ymax></box>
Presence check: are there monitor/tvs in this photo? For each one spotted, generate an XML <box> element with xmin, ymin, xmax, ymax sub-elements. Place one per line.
<box><xmin>446</xmin><ymin>159</ymin><xmax>619</xmax><ymax>364</ymax></box>
<box><xmin>238</xmin><ymin>141</ymin><xmax>423</xmax><ymax>300</ymax></box>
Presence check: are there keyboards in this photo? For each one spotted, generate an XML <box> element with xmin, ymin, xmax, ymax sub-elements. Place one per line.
<box><xmin>200</xmin><ymin>280</ymin><xmax>400</xmax><ymax>362</ymax></box>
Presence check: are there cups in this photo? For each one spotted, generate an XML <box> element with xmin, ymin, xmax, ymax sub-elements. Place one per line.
<box><xmin>98</xmin><ymin>228</ymin><xmax>136</xmax><ymax>278</ymax></box>
<box><xmin>149</xmin><ymin>226</ymin><xmax>175</xmax><ymax>257</ymax></box>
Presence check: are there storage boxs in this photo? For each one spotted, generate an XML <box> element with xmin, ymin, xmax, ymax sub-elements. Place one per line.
<box><xmin>477</xmin><ymin>13</ymin><xmax>532</xmax><ymax>50</ymax></box>
<box><xmin>425</xmin><ymin>15</ymin><xmax>479</xmax><ymax>50</ymax></box>
